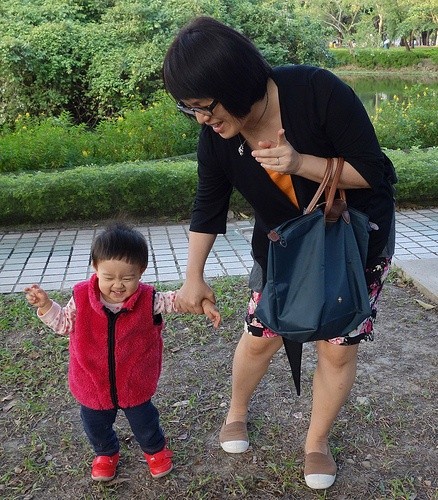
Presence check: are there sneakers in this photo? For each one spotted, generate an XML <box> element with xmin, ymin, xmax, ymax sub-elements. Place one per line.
<box><xmin>303</xmin><ymin>444</ymin><xmax>336</xmax><ymax>489</ymax></box>
<box><xmin>91</xmin><ymin>452</ymin><xmax>119</xmax><ymax>480</ymax></box>
<box><xmin>142</xmin><ymin>444</ymin><xmax>177</xmax><ymax>479</ymax></box>
<box><xmin>220</xmin><ymin>418</ymin><xmax>249</xmax><ymax>454</ymax></box>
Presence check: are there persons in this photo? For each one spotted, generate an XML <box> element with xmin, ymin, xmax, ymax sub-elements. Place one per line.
<box><xmin>161</xmin><ymin>18</ymin><xmax>398</xmax><ymax>489</ymax></box>
<box><xmin>24</xmin><ymin>222</ymin><xmax>220</xmax><ymax>481</ymax></box>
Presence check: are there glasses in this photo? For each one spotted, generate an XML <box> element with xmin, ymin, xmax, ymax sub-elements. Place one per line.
<box><xmin>176</xmin><ymin>99</ymin><xmax>217</xmax><ymax>116</ymax></box>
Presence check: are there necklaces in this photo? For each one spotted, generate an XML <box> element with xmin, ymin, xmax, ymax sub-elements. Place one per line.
<box><xmin>237</xmin><ymin>89</ymin><xmax>269</xmax><ymax>156</ymax></box>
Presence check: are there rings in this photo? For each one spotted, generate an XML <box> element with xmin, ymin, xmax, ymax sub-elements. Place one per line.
<box><xmin>276</xmin><ymin>157</ymin><xmax>280</xmax><ymax>165</ymax></box>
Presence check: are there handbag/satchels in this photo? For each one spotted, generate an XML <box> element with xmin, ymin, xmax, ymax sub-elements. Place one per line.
<box><xmin>255</xmin><ymin>157</ymin><xmax>370</xmax><ymax>342</ymax></box>
